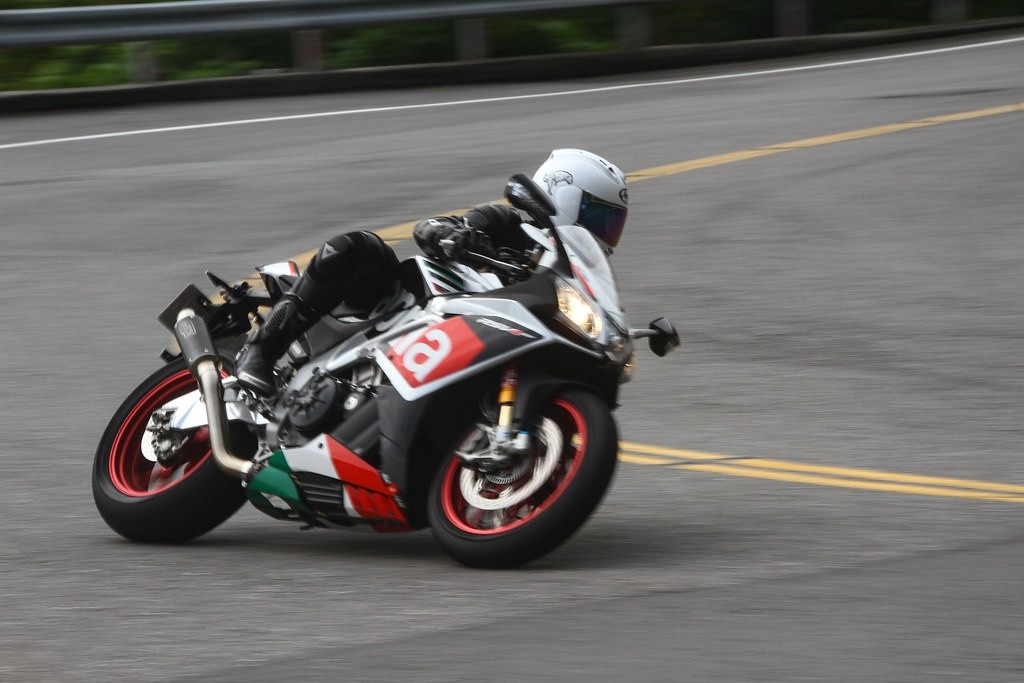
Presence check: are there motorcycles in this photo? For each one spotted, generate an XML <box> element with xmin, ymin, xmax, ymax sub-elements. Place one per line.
<box><xmin>91</xmin><ymin>237</ymin><xmax>681</xmax><ymax>572</ymax></box>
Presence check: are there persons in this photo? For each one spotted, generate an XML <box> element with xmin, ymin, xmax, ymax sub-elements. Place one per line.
<box><xmin>234</xmin><ymin>150</ymin><xmax>627</xmax><ymax>395</ymax></box>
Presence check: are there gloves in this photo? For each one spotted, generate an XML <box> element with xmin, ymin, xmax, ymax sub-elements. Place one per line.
<box><xmin>431</xmin><ymin>224</ymin><xmax>498</xmax><ymax>267</ymax></box>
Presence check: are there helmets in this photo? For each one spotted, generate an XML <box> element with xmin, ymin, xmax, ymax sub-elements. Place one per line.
<box><xmin>533</xmin><ymin>148</ymin><xmax>628</xmax><ymax>258</ymax></box>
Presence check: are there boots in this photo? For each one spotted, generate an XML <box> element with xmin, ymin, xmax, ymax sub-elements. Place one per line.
<box><xmin>235</xmin><ymin>291</ymin><xmax>316</xmax><ymax>393</ymax></box>
